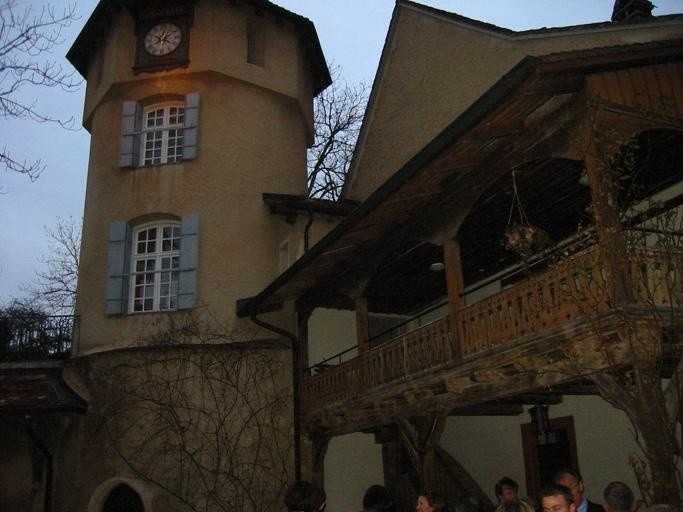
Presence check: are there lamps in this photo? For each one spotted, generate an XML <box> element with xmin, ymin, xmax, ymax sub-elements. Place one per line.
<box><xmin>526</xmin><ymin>398</ymin><xmax>554</xmax><ymax>436</ymax></box>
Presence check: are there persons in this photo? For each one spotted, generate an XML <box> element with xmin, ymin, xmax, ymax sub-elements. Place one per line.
<box><xmin>602</xmin><ymin>482</ymin><xmax>637</xmax><ymax>512</ymax></box>
<box><xmin>493</xmin><ymin>477</ymin><xmax>535</xmax><ymax>512</ymax></box>
<box><xmin>416</xmin><ymin>494</ymin><xmax>445</xmax><ymax>511</ymax></box>
<box><xmin>537</xmin><ymin>483</ymin><xmax>578</xmax><ymax>512</ymax></box>
<box><xmin>551</xmin><ymin>469</ymin><xmax>606</xmax><ymax>512</ymax></box>
<box><xmin>363</xmin><ymin>485</ymin><xmax>397</xmax><ymax>512</ymax></box>
<box><xmin>284</xmin><ymin>481</ymin><xmax>325</xmax><ymax>512</ymax></box>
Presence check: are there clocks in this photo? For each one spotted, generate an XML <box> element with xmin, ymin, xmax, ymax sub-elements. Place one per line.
<box><xmin>142</xmin><ymin>20</ymin><xmax>186</xmax><ymax>58</ymax></box>
